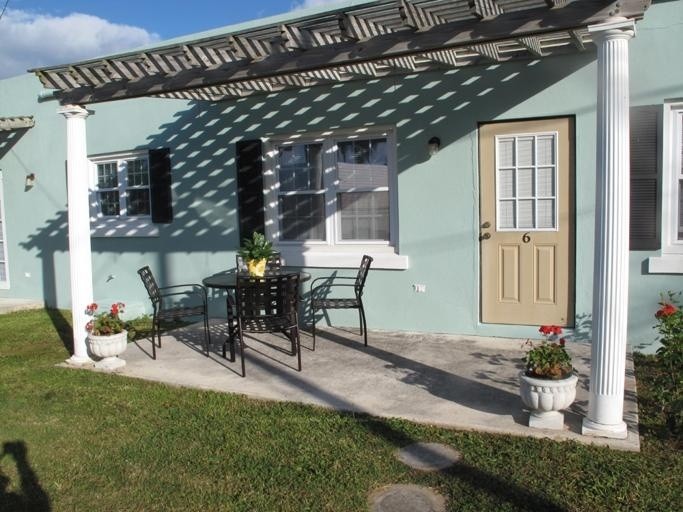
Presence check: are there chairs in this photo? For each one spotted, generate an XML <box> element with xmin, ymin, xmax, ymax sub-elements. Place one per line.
<box><xmin>136</xmin><ymin>250</ymin><xmax>373</xmax><ymax>376</ymax></box>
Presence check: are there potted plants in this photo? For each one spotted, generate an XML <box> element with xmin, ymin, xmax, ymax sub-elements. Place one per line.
<box><xmin>236</xmin><ymin>232</ymin><xmax>276</xmax><ymax>278</ymax></box>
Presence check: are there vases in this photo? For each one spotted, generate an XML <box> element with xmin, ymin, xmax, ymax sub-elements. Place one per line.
<box><xmin>519</xmin><ymin>370</ymin><xmax>579</xmax><ymax>432</ymax></box>
<box><xmin>88</xmin><ymin>330</ymin><xmax>127</xmax><ymax>371</ymax></box>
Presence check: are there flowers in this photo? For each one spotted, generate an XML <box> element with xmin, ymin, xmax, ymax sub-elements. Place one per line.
<box><xmin>84</xmin><ymin>302</ymin><xmax>125</xmax><ymax>336</ymax></box>
<box><xmin>524</xmin><ymin>325</ymin><xmax>573</xmax><ymax>376</ymax></box>
